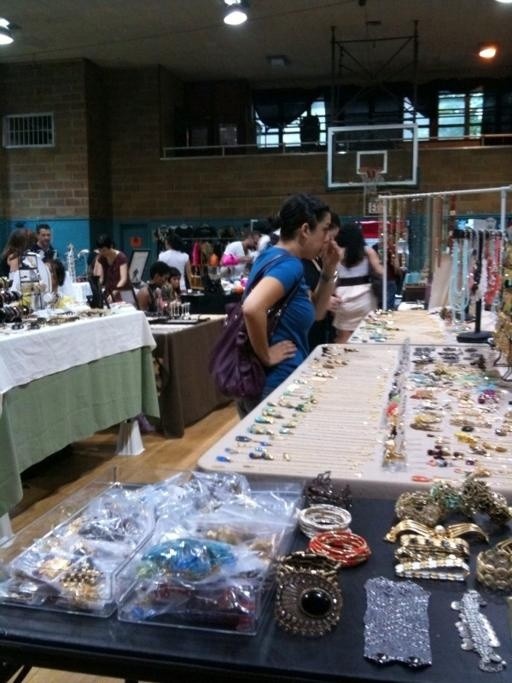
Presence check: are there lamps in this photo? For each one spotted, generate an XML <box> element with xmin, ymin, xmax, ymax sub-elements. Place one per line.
<box><xmin>222</xmin><ymin>0</ymin><xmax>248</xmax><ymax>25</ymax></box>
<box><xmin>0</xmin><ymin>16</ymin><xmax>23</xmax><ymax>45</ymax></box>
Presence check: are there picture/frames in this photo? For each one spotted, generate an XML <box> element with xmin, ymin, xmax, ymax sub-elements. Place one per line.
<box><xmin>128</xmin><ymin>250</ymin><xmax>151</xmax><ymax>286</ymax></box>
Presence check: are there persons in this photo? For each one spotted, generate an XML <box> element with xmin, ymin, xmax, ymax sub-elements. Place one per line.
<box><xmin>0</xmin><ymin>223</ymin><xmax>276</xmax><ymax>316</ymax></box>
<box><xmin>302</xmin><ymin>217</ymin><xmax>407</xmax><ymax>343</ymax></box>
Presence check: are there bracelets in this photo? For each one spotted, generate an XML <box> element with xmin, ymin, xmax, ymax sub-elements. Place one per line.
<box><xmin>297</xmin><ymin>504</ymin><xmax>373</xmax><ymax>568</ymax></box>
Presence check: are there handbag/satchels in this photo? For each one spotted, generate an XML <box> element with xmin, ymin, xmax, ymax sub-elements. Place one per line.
<box><xmin>207</xmin><ymin>304</ymin><xmax>283</xmax><ymax>400</ymax></box>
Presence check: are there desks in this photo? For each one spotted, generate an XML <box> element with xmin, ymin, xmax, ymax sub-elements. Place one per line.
<box><xmin>1</xmin><ymin>310</ymin><xmax>144</xmax><ymax>545</ymax></box>
<box><xmin>197</xmin><ymin>343</ymin><xmax>512</xmax><ymax>501</ymax></box>
<box><xmin>0</xmin><ymin>465</ymin><xmax>512</xmax><ymax>683</ymax></box>
<box><xmin>346</xmin><ymin>309</ymin><xmax>490</xmax><ymax>347</ymax></box>
<box><xmin>146</xmin><ymin>314</ymin><xmax>232</xmax><ymax>439</ymax></box>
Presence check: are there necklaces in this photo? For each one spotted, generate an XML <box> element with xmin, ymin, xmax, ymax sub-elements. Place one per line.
<box><xmin>216</xmin><ymin>345</ymin><xmax>404</xmax><ymax>483</ymax></box>
<box><xmin>450</xmin><ymin>226</ymin><xmax>506</xmax><ymax>325</ymax></box>
